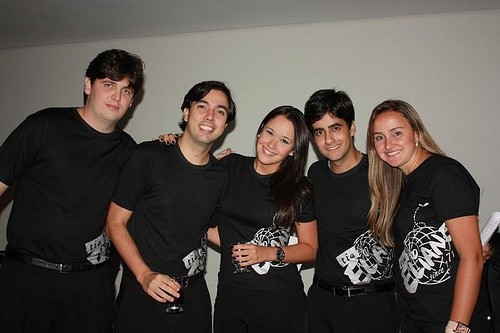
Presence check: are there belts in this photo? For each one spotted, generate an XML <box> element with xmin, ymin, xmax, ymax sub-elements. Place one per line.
<box><xmin>5</xmin><ymin>247</ymin><xmax>98</xmax><ymax>273</ymax></box>
<box><xmin>313</xmin><ymin>275</ymin><xmax>396</xmax><ymax>297</ymax></box>
<box><xmin>172</xmin><ymin>271</ymin><xmax>206</xmax><ymax>285</ymax></box>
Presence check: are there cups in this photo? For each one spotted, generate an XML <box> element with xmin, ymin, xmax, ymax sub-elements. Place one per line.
<box><xmin>231</xmin><ymin>241</ymin><xmax>250</xmax><ymax>273</ymax></box>
<box><xmin>163</xmin><ymin>277</ymin><xmax>185</xmax><ymax>313</ymax></box>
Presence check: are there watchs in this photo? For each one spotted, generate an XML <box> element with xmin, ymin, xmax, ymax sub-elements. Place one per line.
<box><xmin>445</xmin><ymin>319</ymin><xmax>471</xmax><ymax>333</ymax></box>
<box><xmin>276</xmin><ymin>245</ymin><xmax>285</xmax><ymax>264</ymax></box>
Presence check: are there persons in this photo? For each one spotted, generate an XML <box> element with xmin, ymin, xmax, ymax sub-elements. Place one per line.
<box><xmin>364</xmin><ymin>99</ymin><xmax>484</xmax><ymax>333</ymax></box>
<box><xmin>158</xmin><ymin>105</ymin><xmax>318</xmax><ymax>333</ymax></box>
<box><xmin>104</xmin><ymin>80</ymin><xmax>227</xmax><ymax>333</ymax></box>
<box><xmin>304</xmin><ymin>87</ymin><xmax>403</xmax><ymax>333</ymax></box>
<box><xmin>469</xmin><ymin>211</ymin><xmax>500</xmax><ymax>333</ymax></box>
<box><xmin>0</xmin><ymin>49</ymin><xmax>146</xmax><ymax>333</ymax></box>
